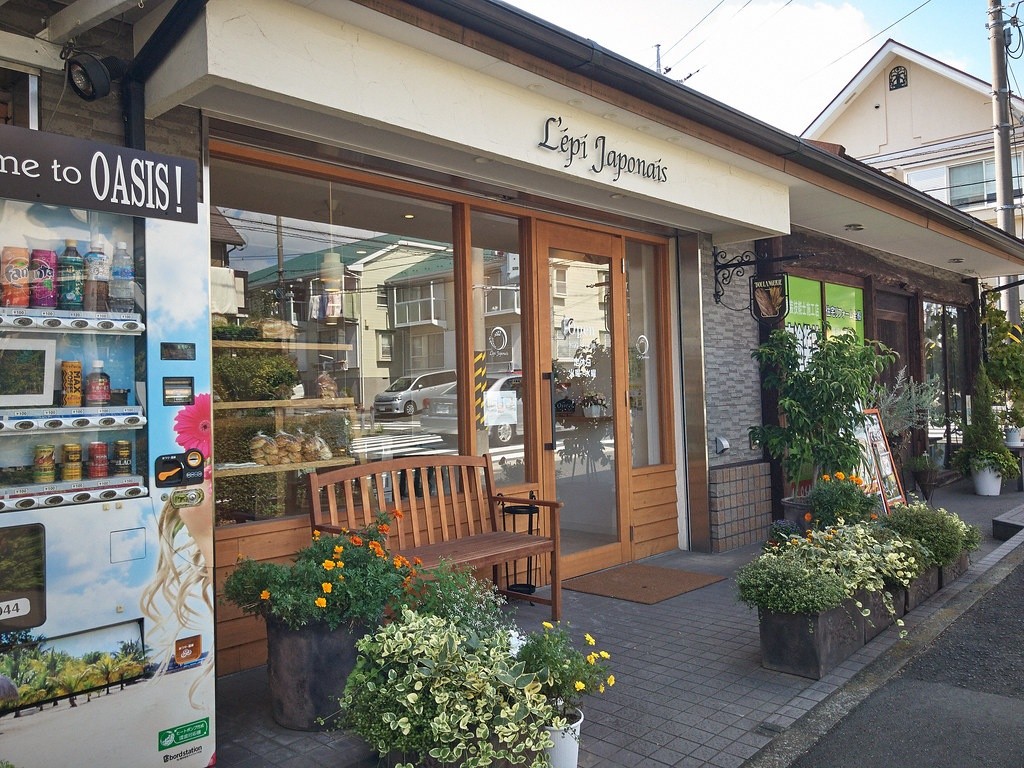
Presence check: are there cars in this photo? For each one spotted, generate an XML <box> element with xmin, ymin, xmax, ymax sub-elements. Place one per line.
<box><xmin>420</xmin><ymin>371</ymin><xmax>583</xmax><ymax>448</ymax></box>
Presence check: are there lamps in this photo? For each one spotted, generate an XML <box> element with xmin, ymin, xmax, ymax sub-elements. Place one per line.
<box><xmin>321</xmin><ymin>182</ymin><xmax>345</xmax><ymax>292</ymax></box>
<box><xmin>64</xmin><ymin>52</ymin><xmax>125</xmax><ymax>101</ymax></box>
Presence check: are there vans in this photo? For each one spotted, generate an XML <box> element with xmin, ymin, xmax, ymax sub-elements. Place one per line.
<box><xmin>373</xmin><ymin>369</ymin><xmax>457</xmax><ymax>415</ymax></box>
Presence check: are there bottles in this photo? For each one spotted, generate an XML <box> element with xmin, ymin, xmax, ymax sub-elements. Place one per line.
<box><xmin>56</xmin><ymin>239</ymin><xmax>136</xmax><ymax>314</ymax></box>
<box><xmin>84</xmin><ymin>361</ymin><xmax>112</xmax><ymax>407</ymax></box>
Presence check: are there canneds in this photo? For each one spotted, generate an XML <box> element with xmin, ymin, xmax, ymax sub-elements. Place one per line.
<box><xmin>88</xmin><ymin>442</ymin><xmax>109</xmax><ymax>478</ymax></box>
<box><xmin>0</xmin><ymin>246</ymin><xmax>30</xmax><ymax>308</ymax></box>
<box><xmin>34</xmin><ymin>445</ymin><xmax>56</xmax><ymax>484</ymax></box>
<box><xmin>113</xmin><ymin>439</ymin><xmax>133</xmax><ymax>476</ymax></box>
<box><xmin>61</xmin><ymin>360</ymin><xmax>82</xmax><ymax>407</ymax></box>
<box><xmin>29</xmin><ymin>249</ymin><xmax>58</xmax><ymax>310</ymax></box>
<box><xmin>61</xmin><ymin>444</ymin><xmax>83</xmax><ymax>482</ymax></box>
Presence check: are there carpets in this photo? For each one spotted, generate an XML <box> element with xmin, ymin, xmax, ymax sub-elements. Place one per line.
<box><xmin>560</xmin><ymin>564</ymin><xmax>728</xmax><ymax>605</ymax></box>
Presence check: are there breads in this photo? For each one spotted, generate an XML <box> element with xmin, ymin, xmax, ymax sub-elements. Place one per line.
<box><xmin>315</xmin><ymin>377</ymin><xmax>337</xmax><ymax>399</ymax></box>
<box><xmin>249</xmin><ymin>434</ymin><xmax>332</xmax><ymax>466</ymax></box>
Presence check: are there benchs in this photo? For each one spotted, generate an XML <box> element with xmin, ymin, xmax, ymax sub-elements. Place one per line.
<box><xmin>307</xmin><ymin>452</ymin><xmax>563</xmax><ymax>626</ymax></box>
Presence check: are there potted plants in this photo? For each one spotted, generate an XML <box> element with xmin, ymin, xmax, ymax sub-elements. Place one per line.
<box><xmin>999</xmin><ymin>409</ymin><xmax>1022</xmax><ymax>447</ymax></box>
<box><xmin>312</xmin><ymin>605</ymin><xmax>571</xmax><ymax>768</ymax></box>
<box><xmin>833</xmin><ymin>524</ymin><xmax>942</xmax><ymax>616</ymax></box>
<box><xmin>910</xmin><ymin>455</ymin><xmax>939</xmax><ymax>500</ymax></box>
<box><xmin>784</xmin><ymin>524</ymin><xmax>906</xmax><ymax>648</ymax></box>
<box><xmin>879</xmin><ymin>495</ymin><xmax>984</xmax><ymax>588</ymax></box>
<box><xmin>738</xmin><ymin>547</ymin><xmax>866</xmax><ymax>681</ymax></box>
<box><xmin>952</xmin><ymin>401</ymin><xmax>1021</xmax><ymax>495</ymax></box>
<box><xmin>746</xmin><ymin>320</ymin><xmax>901</xmax><ymax>529</ymax></box>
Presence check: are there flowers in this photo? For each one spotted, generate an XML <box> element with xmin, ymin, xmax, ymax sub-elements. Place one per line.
<box><xmin>226</xmin><ymin>510</ymin><xmax>422</xmax><ymax>629</ymax></box>
<box><xmin>579</xmin><ymin>391</ymin><xmax>607</xmax><ymax>407</ymax></box>
<box><xmin>517</xmin><ymin>620</ymin><xmax>616</xmax><ymax>709</ymax></box>
<box><xmin>804</xmin><ymin>470</ymin><xmax>883</xmax><ymax>528</ymax></box>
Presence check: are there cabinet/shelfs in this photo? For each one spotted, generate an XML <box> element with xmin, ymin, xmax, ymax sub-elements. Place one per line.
<box><xmin>212</xmin><ymin>339</ymin><xmax>355</xmax><ymax>519</ymax></box>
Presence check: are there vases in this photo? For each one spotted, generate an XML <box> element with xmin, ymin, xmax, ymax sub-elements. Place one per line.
<box><xmin>532</xmin><ymin>712</ymin><xmax>585</xmax><ymax>767</ymax></box>
<box><xmin>583</xmin><ymin>405</ymin><xmax>605</xmax><ymax>418</ymax></box>
<box><xmin>269</xmin><ymin>622</ymin><xmax>362</xmax><ymax>730</ymax></box>
